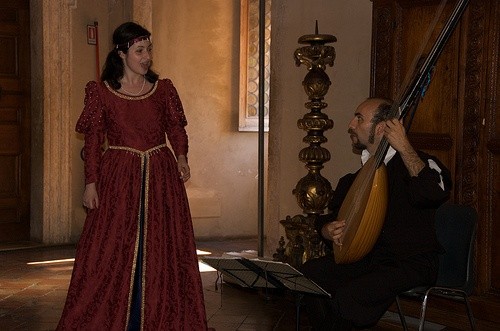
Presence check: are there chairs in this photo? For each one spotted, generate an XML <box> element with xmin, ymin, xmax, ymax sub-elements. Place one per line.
<box><xmin>394</xmin><ymin>203</ymin><xmax>479</xmax><ymax>331</ymax></box>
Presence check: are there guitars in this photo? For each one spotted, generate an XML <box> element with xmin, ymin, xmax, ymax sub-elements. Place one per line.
<box><xmin>332</xmin><ymin>0</ymin><xmax>476</xmax><ymax>265</ymax></box>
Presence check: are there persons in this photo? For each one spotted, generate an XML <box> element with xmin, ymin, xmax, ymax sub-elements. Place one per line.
<box><xmin>81</xmin><ymin>23</ymin><xmax>191</xmax><ymax>331</ymax></box>
<box><xmin>298</xmin><ymin>94</ymin><xmax>454</xmax><ymax>331</ymax></box>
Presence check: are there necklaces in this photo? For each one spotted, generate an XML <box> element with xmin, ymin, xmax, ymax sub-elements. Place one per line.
<box><xmin>121</xmin><ymin>76</ymin><xmax>146</xmax><ymax>96</ymax></box>
